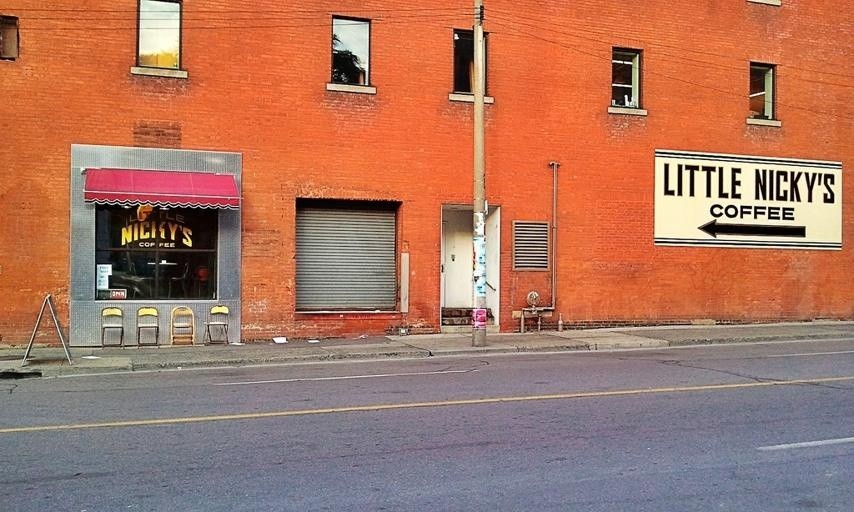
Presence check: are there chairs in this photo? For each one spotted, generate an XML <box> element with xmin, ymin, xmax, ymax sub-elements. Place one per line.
<box><xmin>99</xmin><ymin>306</ymin><xmax>125</xmax><ymax>348</ymax></box>
<box><xmin>170</xmin><ymin>262</ymin><xmax>188</xmax><ymax>298</ymax></box>
<box><xmin>205</xmin><ymin>305</ymin><xmax>229</xmax><ymax>344</ymax></box>
<box><xmin>137</xmin><ymin>307</ymin><xmax>158</xmax><ymax>347</ymax></box>
<box><xmin>131</xmin><ymin>263</ymin><xmax>153</xmax><ymax>298</ymax></box>
<box><xmin>193</xmin><ymin>265</ymin><xmax>208</xmax><ymax>297</ymax></box>
<box><xmin>171</xmin><ymin>306</ymin><xmax>193</xmax><ymax>345</ymax></box>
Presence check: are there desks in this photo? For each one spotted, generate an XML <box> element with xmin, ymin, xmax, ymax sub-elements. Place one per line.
<box><xmin>147</xmin><ymin>261</ymin><xmax>178</xmax><ymax>296</ymax></box>
